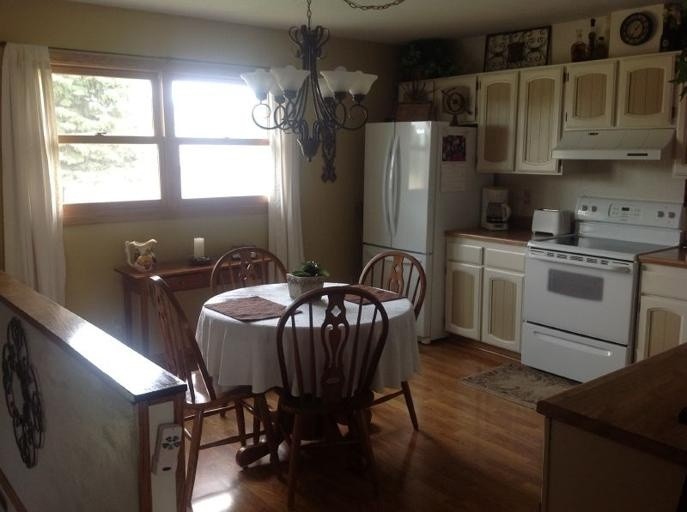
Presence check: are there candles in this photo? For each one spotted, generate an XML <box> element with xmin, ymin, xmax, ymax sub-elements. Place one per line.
<box><xmin>192</xmin><ymin>234</ymin><xmax>206</xmax><ymax>258</ymax></box>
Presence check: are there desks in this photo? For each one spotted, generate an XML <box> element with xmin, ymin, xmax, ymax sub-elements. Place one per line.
<box><xmin>536</xmin><ymin>342</ymin><xmax>687</xmax><ymax>512</ymax></box>
<box><xmin>114</xmin><ymin>253</ymin><xmax>274</xmax><ymax>356</ymax></box>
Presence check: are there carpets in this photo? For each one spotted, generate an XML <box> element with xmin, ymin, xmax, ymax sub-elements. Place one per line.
<box><xmin>458</xmin><ymin>360</ymin><xmax>578</xmax><ymax>411</ymax></box>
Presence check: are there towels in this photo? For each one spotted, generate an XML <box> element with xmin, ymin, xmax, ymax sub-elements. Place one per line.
<box><xmin>343</xmin><ymin>284</ymin><xmax>409</xmax><ymax>307</ymax></box>
<box><xmin>203</xmin><ymin>293</ymin><xmax>301</xmax><ymax>323</ymax></box>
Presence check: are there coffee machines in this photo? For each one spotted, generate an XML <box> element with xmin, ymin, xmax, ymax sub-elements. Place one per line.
<box><xmin>479</xmin><ymin>174</ymin><xmax>511</xmax><ymax>232</ymax></box>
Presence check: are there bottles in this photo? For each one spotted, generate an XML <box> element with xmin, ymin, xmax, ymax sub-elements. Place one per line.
<box><xmin>570</xmin><ymin>17</ymin><xmax>605</xmax><ymax>62</ymax></box>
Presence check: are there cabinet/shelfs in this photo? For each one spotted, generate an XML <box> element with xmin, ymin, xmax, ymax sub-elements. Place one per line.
<box><xmin>628</xmin><ymin>264</ymin><xmax>686</xmax><ymax>364</ymax></box>
<box><xmin>559</xmin><ymin>50</ymin><xmax>676</xmax><ymax>127</ymax></box>
<box><xmin>443</xmin><ymin>233</ymin><xmax>528</xmax><ymax>356</ymax></box>
<box><xmin>478</xmin><ymin>67</ymin><xmax>605</xmax><ymax>177</ymax></box>
<box><xmin>395</xmin><ymin>73</ymin><xmax>479</xmax><ymax>128</ymax></box>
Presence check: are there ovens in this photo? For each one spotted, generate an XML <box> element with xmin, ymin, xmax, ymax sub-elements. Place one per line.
<box><xmin>523</xmin><ymin>247</ymin><xmax>633</xmax><ymax>347</ymax></box>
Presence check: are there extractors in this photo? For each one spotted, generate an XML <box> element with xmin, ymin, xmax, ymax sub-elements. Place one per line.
<box><xmin>549</xmin><ymin>128</ymin><xmax>676</xmax><ymax>162</ymax></box>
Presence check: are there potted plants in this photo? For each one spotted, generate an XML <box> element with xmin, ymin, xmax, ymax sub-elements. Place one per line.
<box><xmin>286</xmin><ymin>262</ymin><xmax>328</xmax><ymax>302</ymax></box>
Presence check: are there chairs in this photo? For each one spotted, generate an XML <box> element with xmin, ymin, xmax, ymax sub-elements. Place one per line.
<box><xmin>146</xmin><ymin>273</ymin><xmax>284</xmax><ymax>511</ymax></box>
<box><xmin>212</xmin><ymin>247</ymin><xmax>291</xmax><ymax>460</ymax></box>
<box><xmin>270</xmin><ymin>285</ymin><xmax>389</xmax><ymax>512</ymax></box>
<box><xmin>359</xmin><ymin>249</ymin><xmax>427</xmax><ymax>432</ymax></box>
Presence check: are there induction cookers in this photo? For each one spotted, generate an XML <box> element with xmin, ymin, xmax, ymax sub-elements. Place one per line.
<box><xmin>528</xmin><ymin>235</ymin><xmax>671</xmax><ymax>260</ymax></box>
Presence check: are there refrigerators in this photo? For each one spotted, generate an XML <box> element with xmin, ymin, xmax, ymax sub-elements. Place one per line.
<box><xmin>360</xmin><ymin>118</ymin><xmax>495</xmax><ymax>349</ymax></box>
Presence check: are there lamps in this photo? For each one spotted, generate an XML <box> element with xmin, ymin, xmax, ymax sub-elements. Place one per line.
<box><xmin>241</xmin><ymin>2</ymin><xmax>378</xmax><ymax>185</ymax></box>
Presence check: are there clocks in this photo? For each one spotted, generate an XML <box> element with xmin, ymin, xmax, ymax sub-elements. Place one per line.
<box><xmin>621</xmin><ymin>14</ymin><xmax>652</xmax><ymax>46</ymax></box>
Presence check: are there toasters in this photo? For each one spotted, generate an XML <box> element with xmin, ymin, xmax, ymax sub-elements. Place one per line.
<box><xmin>531</xmin><ymin>207</ymin><xmax>572</xmax><ymax>235</ymax></box>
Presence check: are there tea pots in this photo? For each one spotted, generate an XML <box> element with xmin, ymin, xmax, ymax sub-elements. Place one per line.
<box><xmin>123</xmin><ymin>237</ymin><xmax>162</xmax><ymax>275</ymax></box>
